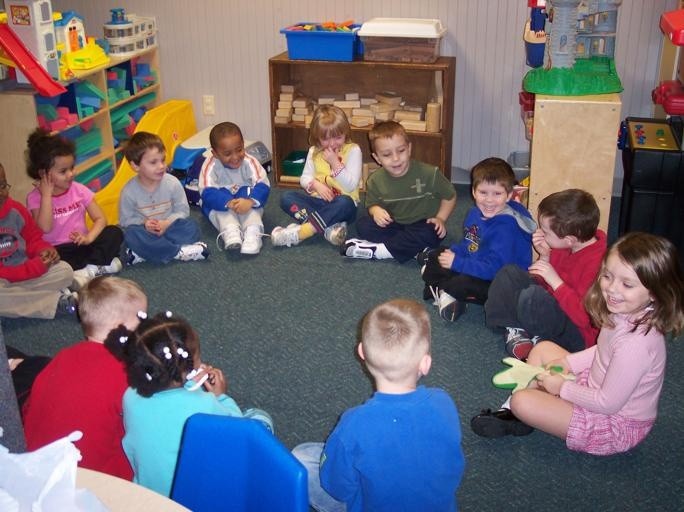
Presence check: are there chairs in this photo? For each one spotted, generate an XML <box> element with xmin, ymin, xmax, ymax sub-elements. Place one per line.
<box><xmin>170</xmin><ymin>412</ymin><xmax>310</xmax><ymax>512</ymax></box>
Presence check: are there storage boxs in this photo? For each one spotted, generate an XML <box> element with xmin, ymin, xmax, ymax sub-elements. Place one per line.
<box><xmin>507</xmin><ymin>151</ymin><xmax>531</xmax><ymax>210</ymax></box>
<box><xmin>358</xmin><ymin>18</ymin><xmax>448</xmax><ymax>63</ymax></box>
<box><xmin>278</xmin><ymin>23</ymin><xmax>358</xmax><ymax>62</ymax></box>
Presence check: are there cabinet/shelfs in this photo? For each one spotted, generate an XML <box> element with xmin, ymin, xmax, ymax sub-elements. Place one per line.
<box><xmin>269</xmin><ymin>59</ymin><xmax>456</xmax><ymax>192</ymax></box>
<box><xmin>0</xmin><ymin>44</ymin><xmax>161</xmax><ymax>214</ymax></box>
<box><xmin>528</xmin><ymin>99</ymin><xmax>622</xmax><ymax>243</ymax></box>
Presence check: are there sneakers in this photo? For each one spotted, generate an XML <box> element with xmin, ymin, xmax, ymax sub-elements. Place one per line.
<box><xmin>56</xmin><ymin>222</ymin><xmax>300</xmax><ymax>317</ymax></box>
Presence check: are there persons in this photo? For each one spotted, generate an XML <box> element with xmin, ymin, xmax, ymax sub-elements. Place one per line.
<box><xmin>271</xmin><ymin>104</ymin><xmax>362</xmax><ymax>249</ymax></box>
<box><xmin>198</xmin><ymin>121</ymin><xmax>271</xmax><ymax>256</ymax></box>
<box><xmin>485</xmin><ymin>188</ymin><xmax>608</xmax><ymax>361</ymax></box>
<box><xmin>25</xmin><ymin>127</ymin><xmax>124</xmax><ymax>293</ymax></box>
<box><xmin>0</xmin><ymin>164</ymin><xmax>81</xmax><ymax>324</ymax></box>
<box><xmin>117</xmin><ymin>131</ymin><xmax>211</xmax><ymax>267</ymax></box>
<box><xmin>103</xmin><ymin>310</ymin><xmax>274</xmax><ymax>497</ymax></box>
<box><xmin>339</xmin><ymin>119</ymin><xmax>457</xmax><ymax>261</ymax></box>
<box><xmin>21</xmin><ymin>275</ymin><xmax>147</xmax><ymax>479</ymax></box>
<box><xmin>417</xmin><ymin>157</ymin><xmax>539</xmax><ymax>322</ymax></box>
<box><xmin>290</xmin><ymin>297</ymin><xmax>466</xmax><ymax>511</ymax></box>
<box><xmin>470</xmin><ymin>229</ymin><xmax>683</xmax><ymax>456</ymax></box>
